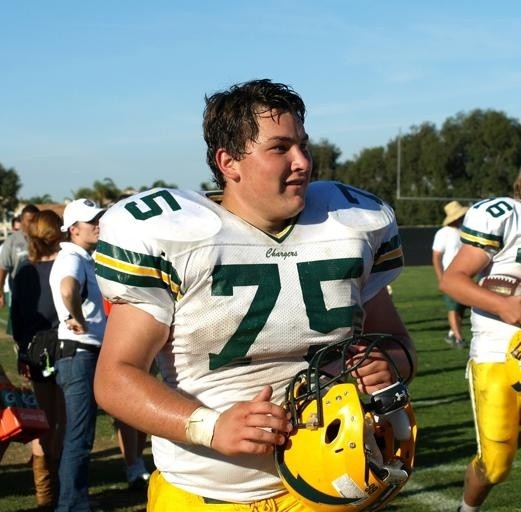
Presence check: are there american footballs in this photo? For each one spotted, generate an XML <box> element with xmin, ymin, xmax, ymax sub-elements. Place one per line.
<box><xmin>476</xmin><ymin>274</ymin><xmax>520</xmax><ymax>297</ymax></box>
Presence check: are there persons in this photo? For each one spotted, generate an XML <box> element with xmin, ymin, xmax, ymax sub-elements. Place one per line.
<box><xmin>431</xmin><ymin>200</ymin><xmax>470</xmax><ymax>350</ymax></box>
<box><xmin>437</xmin><ymin>169</ymin><xmax>521</xmax><ymax>512</ymax></box>
<box><xmin>92</xmin><ymin>78</ymin><xmax>417</xmax><ymax>512</ymax></box>
<box><xmin>0</xmin><ymin>198</ymin><xmax>154</xmax><ymax>510</ymax></box>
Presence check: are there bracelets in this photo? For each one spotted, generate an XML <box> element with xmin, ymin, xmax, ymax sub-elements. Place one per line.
<box><xmin>184</xmin><ymin>405</ymin><xmax>219</xmax><ymax>450</ymax></box>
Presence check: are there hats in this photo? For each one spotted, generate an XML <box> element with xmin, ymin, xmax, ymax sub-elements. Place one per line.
<box><xmin>61</xmin><ymin>198</ymin><xmax>106</xmax><ymax>232</ymax></box>
<box><xmin>442</xmin><ymin>201</ymin><xmax>470</xmax><ymax>226</ymax></box>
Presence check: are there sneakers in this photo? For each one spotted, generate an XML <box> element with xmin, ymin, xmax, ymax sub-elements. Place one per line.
<box><xmin>125</xmin><ymin>459</ymin><xmax>150</xmax><ymax>483</ymax></box>
<box><xmin>443</xmin><ymin>332</ymin><xmax>470</xmax><ymax>352</ymax></box>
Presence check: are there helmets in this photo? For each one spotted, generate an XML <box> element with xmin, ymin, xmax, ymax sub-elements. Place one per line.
<box><xmin>272</xmin><ymin>334</ymin><xmax>421</xmax><ymax>511</ymax></box>
